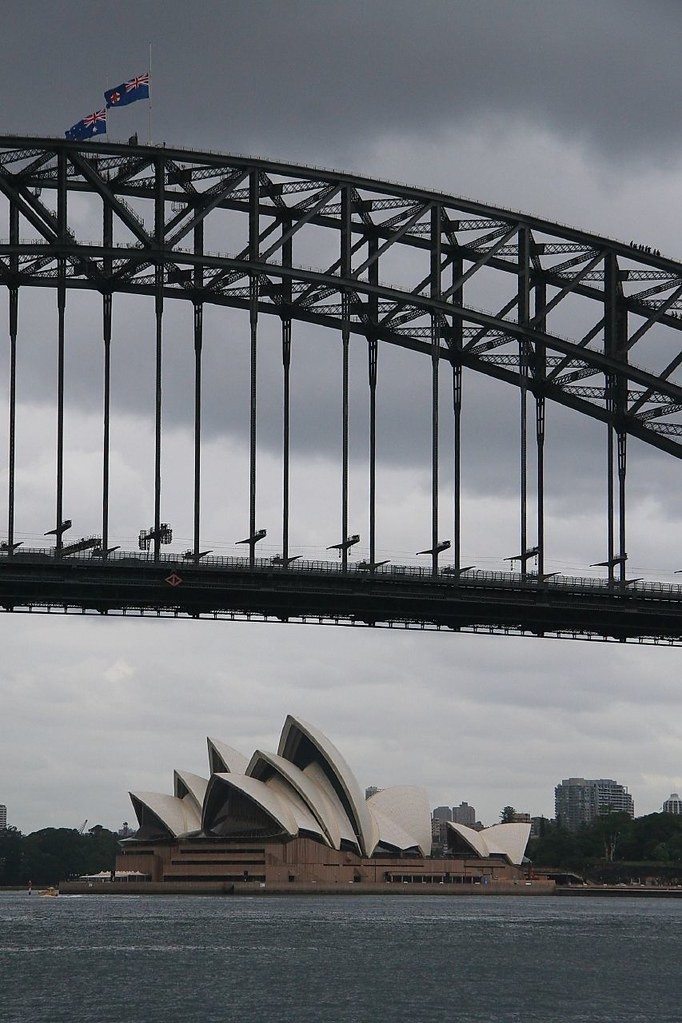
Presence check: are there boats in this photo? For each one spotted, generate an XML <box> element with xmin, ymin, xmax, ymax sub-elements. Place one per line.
<box><xmin>38</xmin><ymin>887</ymin><xmax>58</xmax><ymax>897</ymax></box>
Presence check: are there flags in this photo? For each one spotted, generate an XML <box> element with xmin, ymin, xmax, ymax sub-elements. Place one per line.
<box><xmin>103</xmin><ymin>72</ymin><xmax>149</xmax><ymax>108</ymax></box>
<box><xmin>64</xmin><ymin>111</ymin><xmax>107</xmax><ymax>142</ymax></box>
